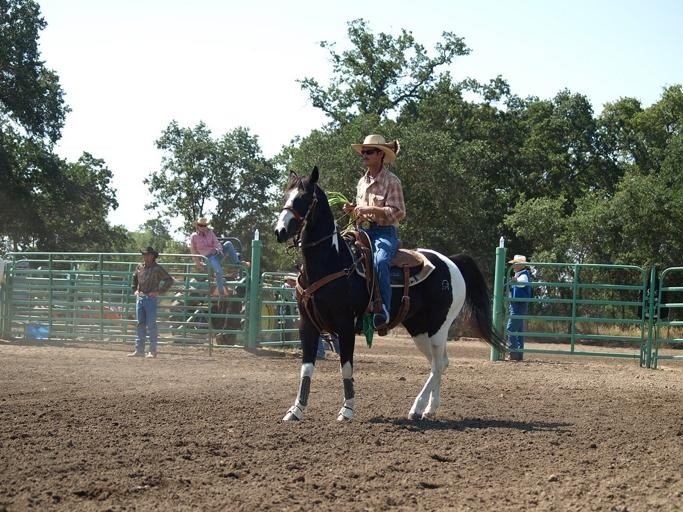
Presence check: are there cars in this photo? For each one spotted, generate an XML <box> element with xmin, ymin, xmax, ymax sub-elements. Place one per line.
<box><xmin>41</xmin><ymin>298</ymin><xmax>130</xmax><ymax>324</ymax></box>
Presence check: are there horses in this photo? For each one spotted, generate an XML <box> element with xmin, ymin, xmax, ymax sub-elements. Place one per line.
<box><xmin>274</xmin><ymin>166</ymin><xmax>518</xmax><ymax>423</ymax></box>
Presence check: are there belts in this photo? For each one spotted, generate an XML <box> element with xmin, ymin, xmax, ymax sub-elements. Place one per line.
<box><xmin>205</xmin><ymin>248</ymin><xmax>218</xmax><ymax>258</ymax></box>
<box><xmin>137</xmin><ymin>290</ymin><xmax>149</xmax><ymax>297</ymax></box>
<box><xmin>357</xmin><ymin>221</ymin><xmax>387</xmax><ymax>230</ymax></box>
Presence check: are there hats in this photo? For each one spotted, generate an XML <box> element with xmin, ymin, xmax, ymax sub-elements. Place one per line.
<box><xmin>351</xmin><ymin>134</ymin><xmax>400</xmax><ymax>164</ymax></box>
<box><xmin>139</xmin><ymin>246</ymin><xmax>159</xmax><ymax>260</ymax></box>
<box><xmin>507</xmin><ymin>254</ymin><xmax>532</xmax><ymax>265</ymax></box>
<box><xmin>282</xmin><ymin>273</ymin><xmax>296</xmax><ymax>282</ymax></box>
<box><xmin>193</xmin><ymin>217</ymin><xmax>212</xmax><ymax>226</ymax></box>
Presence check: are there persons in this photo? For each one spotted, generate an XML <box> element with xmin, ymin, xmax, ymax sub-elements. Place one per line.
<box><xmin>190</xmin><ymin>217</ymin><xmax>245</xmax><ymax>298</ymax></box>
<box><xmin>125</xmin><ymin>247</ymin><xmax>175</xmax><ymax>357</ymax></box>
<box><xmin>169</xmin><ymin>273</ymin><xmax>341</xmax><ymax>359</ymax></box>
<box><xmin>342</xmin><ymin>134</ymin><xmax>407</xmax><ymax>325</ymax></box>
<box><xmin>504</xmin><ymin>255</ymin><xmax>531</xmax><ymax>360</ymax></box>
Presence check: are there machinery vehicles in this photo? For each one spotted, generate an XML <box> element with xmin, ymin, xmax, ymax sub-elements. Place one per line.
<box><xmin>168</xmin><ymin>235</ymin><xmax>298</xmax><ymax>345</ymax></box>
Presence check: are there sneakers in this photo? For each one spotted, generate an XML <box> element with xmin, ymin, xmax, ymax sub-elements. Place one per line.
<box><xmin>143</xmin><ymin>351</ymin><xmax>157</xmax><ymax>358</ymax></box>
<box><xmin>505</xmin><ymin>351</ymin><xmax>524</xmax><ymax>362</ymax></box>
<box><xmin>373</xmin><ymin>312</ymin><xmax>389</xmax><ymax>337</ymax></box>
<box><xmin>126</xmin><ymin>350</ymin><xmax>144</xmax><ymax>357</ymax></box>
